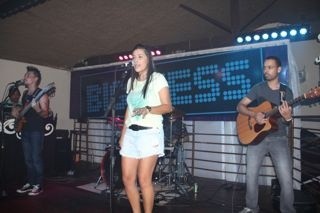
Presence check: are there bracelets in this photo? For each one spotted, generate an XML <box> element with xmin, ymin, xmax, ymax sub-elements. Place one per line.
<box><xmin>146</xmin><ymin>106</ymin><xmax>152</xmax><ymax>113</ymax></box>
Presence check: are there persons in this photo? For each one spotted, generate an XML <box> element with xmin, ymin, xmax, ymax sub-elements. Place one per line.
<box><xmin>119</xmin><ymin>45</ymin><xmax>174</xmax><ymax>213</ymax></box>
<box><xmin>0</xmin><ymin>65</ymin><xmax>49</xmax><ymax>196</ymax></box>
<box><xmin>158</xmin><ymin>109</ymin><xmax>187</xmax><ymax>184</ymax></box>
<box><xmin>236</xmin><ymin>56</ymin><xmax>297</xmax><ymax>213</ymax></box>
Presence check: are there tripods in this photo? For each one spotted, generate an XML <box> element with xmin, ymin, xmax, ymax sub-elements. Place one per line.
<box><xmin>152</xmin><ymin>152</ymin><xmax>184</xmax><ymax>189</ymax></box>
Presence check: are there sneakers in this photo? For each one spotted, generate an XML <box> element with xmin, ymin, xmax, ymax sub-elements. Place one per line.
<box><xmin>16</xmin><ymin>182</ymin><xmax>32</xmax><ymax>194</ymax></box>
<box><xmin>27</xmin><ymin>184</ymin><xmax>43</xmax><ymax>196</ymax></box>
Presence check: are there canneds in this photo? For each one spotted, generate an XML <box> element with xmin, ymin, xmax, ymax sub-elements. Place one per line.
<box><xmin>193</xmin><ymin>182</ymin><xmax>198</xmax><ymax>193</ymax></box>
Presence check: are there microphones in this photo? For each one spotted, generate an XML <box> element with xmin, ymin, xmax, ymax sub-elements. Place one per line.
<box><xmin>127</xmin><ymin>61</ymin><xmax>135</xmax><ymax>68</ymax></box>
<box><xmin>16</xmin><ymin>79</ymin><xmax>23</xmax><ymax>85</ymax></box>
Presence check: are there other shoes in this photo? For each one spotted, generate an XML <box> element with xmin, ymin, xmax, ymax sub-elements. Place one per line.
<box><xmin>240</xmin><ymin>206</ymin><xmax>259</xmax><ymax>212</ymax></box>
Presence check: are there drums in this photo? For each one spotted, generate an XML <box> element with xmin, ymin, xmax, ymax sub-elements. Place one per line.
<box><xmin>101</xmin><ymin>150</ymin><xmax>125</xmax><ymax>191</ymax></box>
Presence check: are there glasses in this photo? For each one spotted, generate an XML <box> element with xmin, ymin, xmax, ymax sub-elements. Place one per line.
<box><xmin>263</xmin><ymin>66</ymin><xmax>274</xmax><ymax>70</ymax></box>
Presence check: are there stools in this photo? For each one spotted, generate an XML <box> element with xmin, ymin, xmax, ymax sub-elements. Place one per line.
<box><xmin>70</xmin><ymin>118</ymin><xmax>89</xmax><ymax>168</ymax></box>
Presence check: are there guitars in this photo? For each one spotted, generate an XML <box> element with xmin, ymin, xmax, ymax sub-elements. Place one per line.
<box><xmin>14</xmin><ymin>82</ymin><xmax>56</xmax><ymax>132</ymax></box>
<box><xmin>236</xmin><ymin>84</ymin><xmax>320</xmax><ymax>145</ymax></box>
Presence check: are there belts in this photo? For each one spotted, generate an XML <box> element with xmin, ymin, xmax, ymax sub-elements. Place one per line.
<box><xmin>128</xmin><ymin>124</ymin><xmax>156</xmax><ymax>131</ymax></box>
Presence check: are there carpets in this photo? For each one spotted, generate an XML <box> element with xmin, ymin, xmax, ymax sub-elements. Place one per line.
<box><xmin>76</xmin><ymin>181</ymin><xmax>194</xmax><ymax>206</ymax></box>
